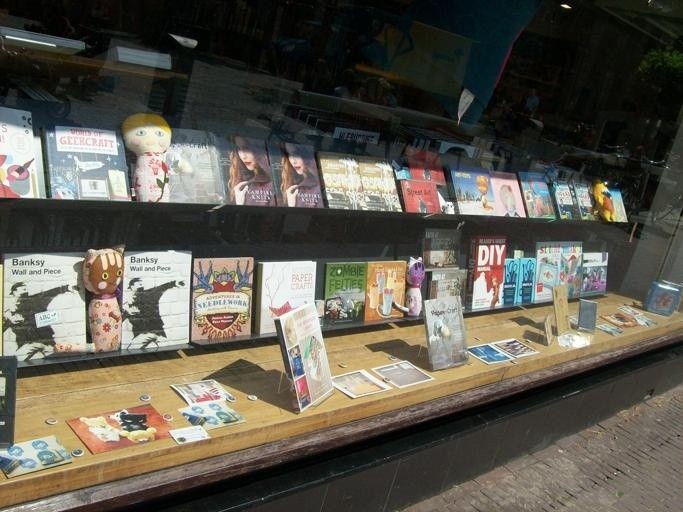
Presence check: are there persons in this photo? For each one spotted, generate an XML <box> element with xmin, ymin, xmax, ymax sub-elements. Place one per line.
<box><xmin>3</xmin><ymin>282</ymin><xmax>80</xmax><ymax>361</ymax></box>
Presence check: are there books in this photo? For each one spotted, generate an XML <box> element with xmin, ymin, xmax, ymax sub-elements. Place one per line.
<box><xmin>2</xmin><ymin>251</ymin><xmax>87</xmax><ymax>360</ymax></box>
<box><xmin>644</xmin><ymin>281</ymin><xmax>680</xmax><ymax>316</ymax></box>
<box><xmin>0</xmin><ymin>106</ymin><xmax>132</xmax><ymax>202</ymax></box>
<box><xmin>423</xmin><ymin>296</ymin><xmax>470</xmax><ymax>370</ymax></box>
<box><xmin>165</xmin><ymin>128</ymin><xmax>628</xmax><ymax>222</ymax></box>
<box><xmin>120</xmin><ymin>235</ymin><xmax>609</xmax><ymax>349</ymax></box>
<box><xmin>275</xmin><ymin>302</ymin><xmax>334</xmax><ymax>413</ymax></box>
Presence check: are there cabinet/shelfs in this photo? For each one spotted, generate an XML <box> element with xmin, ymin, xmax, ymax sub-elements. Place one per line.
<box><xmin>1</xmin><ymin>43</ymin><xmax>627</xmax><ymax>368</ymax></box>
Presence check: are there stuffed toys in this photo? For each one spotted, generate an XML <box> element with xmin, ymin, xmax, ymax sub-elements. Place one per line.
<box><xmin>84</xmin><ymin>244</ymin><xmax>122</xmax><ymax>351</ymax></box>
<box><xmin>120</xmin><ymin>112</ymin><xmax>172</xmax><ymax>204</ymax></box>
